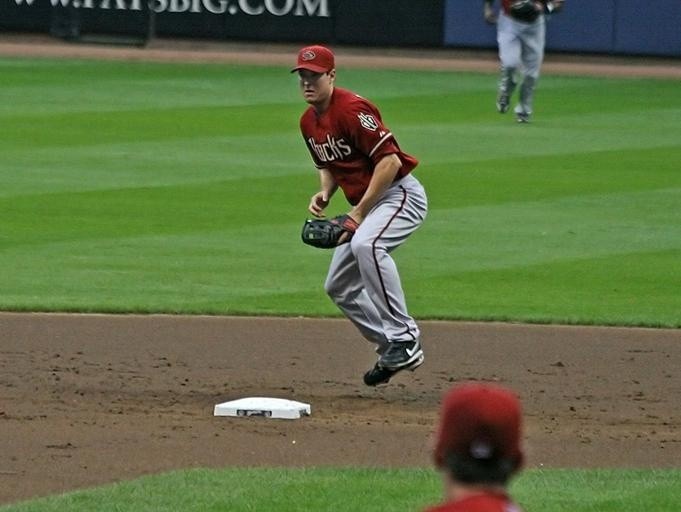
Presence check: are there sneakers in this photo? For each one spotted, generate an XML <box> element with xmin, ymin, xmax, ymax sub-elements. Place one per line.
<box><xmin>496</xmin><ymin>93</ymin><xmax>531</xmax><ymax>121</ymax></box>
<box><xmin>379</xmin><ymin>338</ymin><xmax>423</xmax><ymax>367</ymax></box>
<box><xmin>364</xmin><ymin>354</ymin><xmax>424</xmax><ymax>386</ymax></box>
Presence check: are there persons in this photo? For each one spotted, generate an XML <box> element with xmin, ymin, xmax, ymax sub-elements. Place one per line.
<box><xmin>290</xmin><ymin>44</ymin><xmax>430</xmax><ymax>387</ymax></box>
<box><xmin>483</xmin><ymin>1</ymin><xmax>564</xmax><ymax>124</ymax></box>
<box><xmin>419</xmin><ymin>382</ymin><xmax>531</xmax><ymax>511</ymax></box>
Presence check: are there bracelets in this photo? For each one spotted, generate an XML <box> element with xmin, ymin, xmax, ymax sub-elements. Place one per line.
<box><xmin>544</xmin><ymin>2</ymin><xmax>555</xmax><ymax>14</ymax></box>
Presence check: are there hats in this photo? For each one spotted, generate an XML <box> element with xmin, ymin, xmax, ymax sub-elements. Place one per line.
<box><xmin>436</xmin><ymin>388</ymin><xmax>522</xmax><ymax>469</ymax></box>
<box><xmin>291</xmin><ymin>45</ymin><xmax>335</xmax><ymax>74</ymax></box>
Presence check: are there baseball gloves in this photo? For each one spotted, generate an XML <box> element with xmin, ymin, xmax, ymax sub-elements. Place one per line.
<box><xmin>302</xmin><ymin>214</ymin><xmax>360</xmax><ymax>248</ymax></box>
